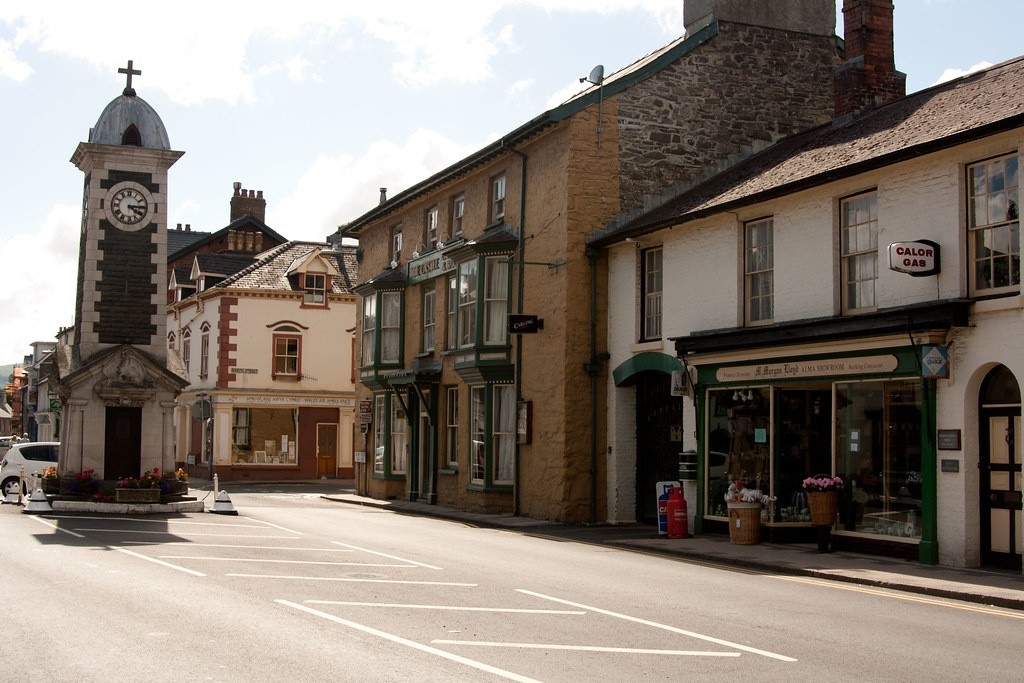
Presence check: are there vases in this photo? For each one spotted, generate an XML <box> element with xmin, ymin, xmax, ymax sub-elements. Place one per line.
<box><xmin>179</xmin><ymin>482</ymin><xmax>189</xmax><ymax>494</ymax></box>
<box><xmin>115</xmin><ymin>488</ymin><xmax>160</xmax><ymax>502</ymax></box>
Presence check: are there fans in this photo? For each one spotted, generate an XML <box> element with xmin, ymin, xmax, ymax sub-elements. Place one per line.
<box><xmin>41</xmin><ymin>478</ymin><xmax>60</xmax><ymax>493</ymax></box>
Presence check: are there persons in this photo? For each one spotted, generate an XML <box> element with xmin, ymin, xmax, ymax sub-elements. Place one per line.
<box><xmin>10</xmin><ymin>433</ymin><xmax>30</xmax><ymax>449</ymax></box>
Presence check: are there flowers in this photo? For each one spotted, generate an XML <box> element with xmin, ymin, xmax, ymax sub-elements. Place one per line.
<box><xmin>43</xmin><ymin>467</ymin><xmax>60</xmax><ymax>480</ymax></box>
<box><xmin>175</xmin><ymin>468</ymin><xmax>188</xmax><ymax>481</ymax></box>
<box><xmin>116</xmin><ymin>470</ymin><xmax>170</xmax><ymax>490</ymax></box>
<box><xmin>802</xmin><ymin>476</ymin><xmax>844</xmax><ymax>494</ymax></box>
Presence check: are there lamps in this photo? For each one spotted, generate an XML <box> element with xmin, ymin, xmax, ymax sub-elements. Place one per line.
<box><xmin>391</xmin><ymin>250</ymin><xmax>402</xmax><ymax>269</ymax></box>
<box><xmin>739</xmin><ymin>389</ymin><xmax>747</xmax><ymax>402</ymax></box>
<box><xmin>747</xmin><ymin>389</ymin><xmax>753</xmax><ymax>400</ymax></box>
<box><xmin>412</xmin><ymin>242</ymin><xmax>427</xmax><ymax>260</ymax></box>
<box><xmin>437</xmin><ymin>232</ymin><xmax>451</xmax><ymax>250</ymax></box>
<box><xmin>733</xmin><ymin>390</ymin><xmax>738</xmax><ymax>401</ymax></box>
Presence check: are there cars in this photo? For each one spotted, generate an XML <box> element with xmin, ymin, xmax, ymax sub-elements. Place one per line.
<box><xmin>0</xmin><ymin>436</ymin><xmax>21</xmax><ymax>460</ymax></box>
<box><xmin>0</xmin><ymin>442</ymin><xmax>60</xmax><ymax>499</ymax></box>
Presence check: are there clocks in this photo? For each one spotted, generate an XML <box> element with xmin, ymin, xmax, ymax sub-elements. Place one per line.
<box><xmin>111</xmin><ymin>188</ymin><xmax>148</xmax><ymax>225</ymax></box>
